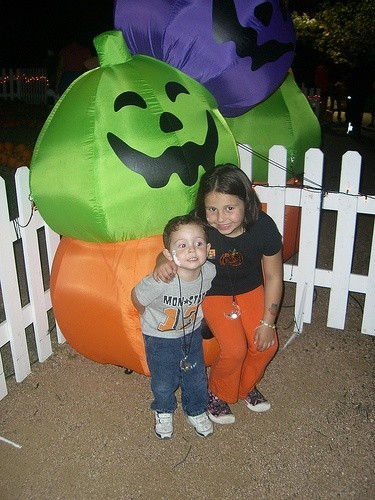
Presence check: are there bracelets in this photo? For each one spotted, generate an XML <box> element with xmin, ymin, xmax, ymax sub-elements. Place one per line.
<box><xmin>260</xmin><ymin>318</ymin><xmax>277</xmax><ymax>330</ymax></box>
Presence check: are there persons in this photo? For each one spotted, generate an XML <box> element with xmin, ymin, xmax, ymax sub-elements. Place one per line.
<box><xmin>343</xmin><ymin>52</ymin><xmax>370</xmax><ymax>133</ymax></box>
<box><xmin>180</xmin><ymin>163</ymin><xmax>284</xmax><ymax>424</ymax></box>
<box><xmin>314</xmin><ymin>56</ymin><xmax>343</xmax><ymax>119</ymax></box>
<box><xmin>45</xmin><ymin>27</ymin><xmax>91</xmax><ymax>109</ymax></box>
<box><xmin>130</xmin><ymin>216</ymin><xmax>216</xmax><ymax>438</ymax></box>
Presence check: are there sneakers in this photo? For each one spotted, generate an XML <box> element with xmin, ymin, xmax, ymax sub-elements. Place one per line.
<box><xmin>206</xmin><ymin>388</ymin><xmax>235</xmax><ymax>424</ymax></box>
<box><xmin>153</xmin><ymin>410</ymin><xmax>174</xmax><ymax>439</ymax></box>
<box><xmin>185</xmin><ymin>412</ymin><xmax>214</xmax><ymax>438</ymax></box>
<box><xmin>243</xmin><ymin>386</ymin><xmax>270</xmax><ymax>412</ymax></box>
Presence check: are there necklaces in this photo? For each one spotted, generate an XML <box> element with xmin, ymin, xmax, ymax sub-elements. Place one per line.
<box><xmin>175</xmin><ymin>270</ymin><xmax>204</xmax><ymax>371</ymax></box>
<box><xmin>207</xmin><ymin>225</ymin><xmax>246</xmax><ymax>321</ymax></box>
